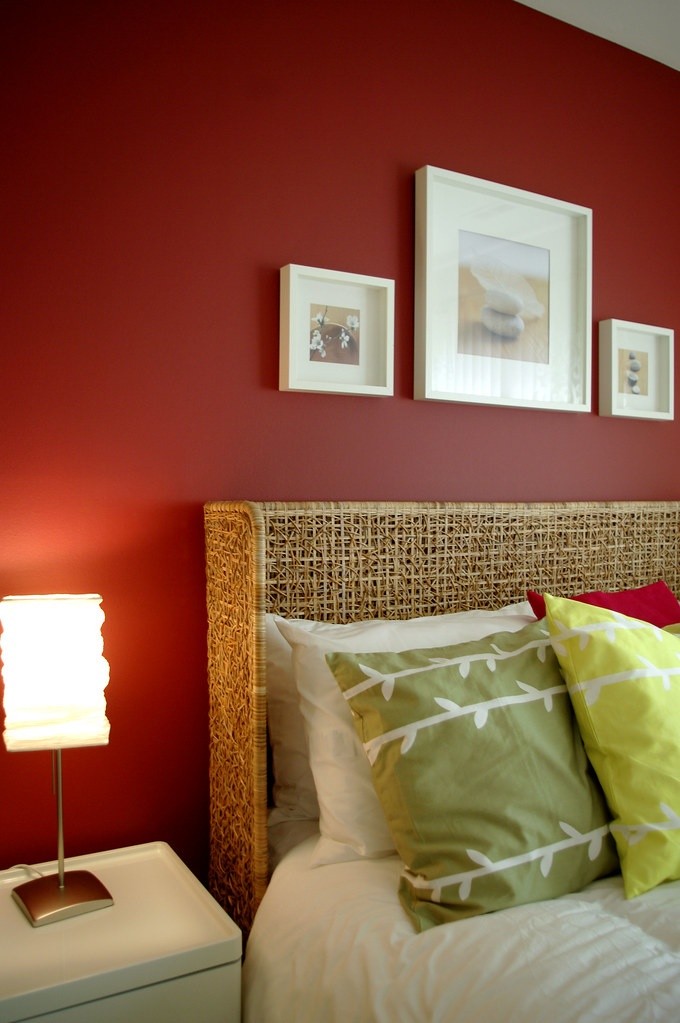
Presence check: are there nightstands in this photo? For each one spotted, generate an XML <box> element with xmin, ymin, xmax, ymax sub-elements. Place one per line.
<box><xmin>0</xmin><ymin>841</ymin><xmax>243</xmax><ymax>1023</ymax></box>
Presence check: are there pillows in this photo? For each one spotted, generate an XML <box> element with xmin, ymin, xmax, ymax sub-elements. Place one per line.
<box><xmin>261</xmin><ymin>597</ymin><xmax>530</xmax><ymax>828</ymax></box>
<box><xmin>274</xmin><ymin>614</ymin><xmax>536</xmax><ymax>871</ymax></box>
<box><xmin>542</xmin><ymin>590</ymin><xmax>680</xmax><ymax>900</ymax></box>
<box><xmin>527</xmin><ymin>578</ymin><xmax>680</xmax><ymax>628</ymax></box>
<box><xmin>326</xmin><ymin>618</ymin><xmax>621</xmax><ymax>933</ymax></box>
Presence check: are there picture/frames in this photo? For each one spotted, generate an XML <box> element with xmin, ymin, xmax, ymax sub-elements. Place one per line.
<box><xmin>278</xmin><ymin>264</ymin><xmax>396</xmax><ymax>398</ymax></box>
<box><xmin>411</xmin><ymin>165</ymin><xmax>594</xmax><ymax>413</ymax></box>
<box><xmin>598</xmin><ymin>318</ymin><xmax>675</xmax><ymax>422</ymax></box>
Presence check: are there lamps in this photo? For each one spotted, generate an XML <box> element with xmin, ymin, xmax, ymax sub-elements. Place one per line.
<box><xmin>0</xmin><ymin>593</ymin><xmax>116</xmax><ymax>926</ymax></box>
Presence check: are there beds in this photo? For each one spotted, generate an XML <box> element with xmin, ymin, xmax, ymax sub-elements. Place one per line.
<box><xmin>208</xmin><ymin>501</ymin><xmax>680</xmax><ymax>1023</ymax></box>
